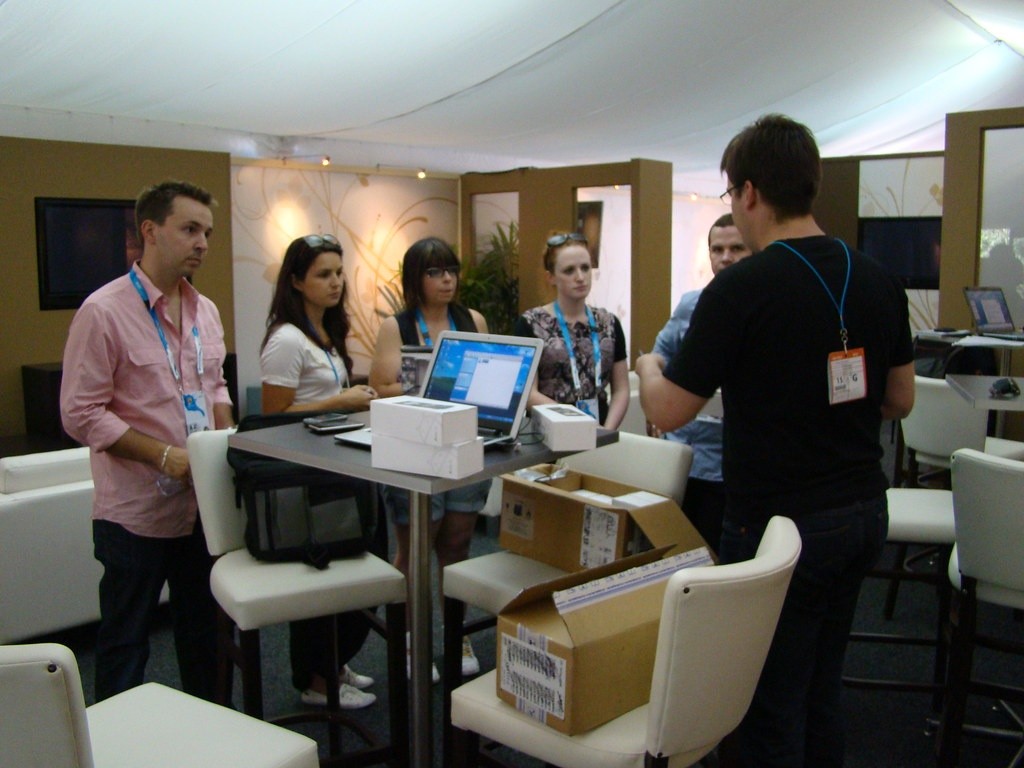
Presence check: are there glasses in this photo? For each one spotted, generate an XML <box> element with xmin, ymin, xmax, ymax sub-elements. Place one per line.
<box><xmin>295</xmin><ymin>235</ymin><xmax>341</xmax><ymax>262</ymax></box>
<box><xmin>720</xmin><ymin>178</ymin><xmax>751</xmax><ymax>205</ymax></box>
<box><xmin>546</xmin><ymin>234</ymin><xmax>587</xmax><ymax>252</ymax></box>
<box><xmin>422</xmin><ymin>265</ymin><xmax>461</xmax><ymax>278</ymax></box>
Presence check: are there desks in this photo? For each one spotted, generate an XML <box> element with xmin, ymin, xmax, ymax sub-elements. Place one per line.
<box><xmin>228</xmin><ymin>409</ymin><xmax>620</xmax><ymax>768</ymax></box>
<box><xmin>913</xmin><ymin>330</ymin><xmax>1023</xmax><ymax>438</ymax></box>
<box><xmin>946</xmin><ymin>374</ymin><xmax>1024</xmax><ymax>413</ymax></box>
<box><xmin>21</xmin><ymin>354</ymin><xmax>239</xmax><ymax>451</ymax></box>
<box><xmin>838</xmin><ymin>489</ymin><xmax>957</xmax><ymax>692</ymax></box>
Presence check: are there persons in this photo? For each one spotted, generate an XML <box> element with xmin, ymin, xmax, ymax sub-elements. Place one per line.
<box><xmin>645</xmin><ymin>212</ymin><xmax>752</xmax><ymax>558</ymax></box>
<box><xmin>259</xmin><ymin>234</ymin><xmax>381</xmax><ymax>710</ymax></box>
<box><xmin>60</xmin><ymin>180</ymin><xmax>237</xmax><ymax>711</ymax></box>
<box><xmin>368</xmin><ymin>236</ymin><xmax>489</xmax><ymax>682</ymax></box>
<box><xmin>506</xmin><ymin>229</ymin><xmax>632</xmax><ymax>432</ymax></box>
<box><xmin>634</xmin><ymin>112</ymin><xmax>916</xmax><ymax>768</ymax></box>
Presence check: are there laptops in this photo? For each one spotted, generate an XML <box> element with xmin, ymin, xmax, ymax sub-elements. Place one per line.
<box><xmin>334</xmin><ymin>330</ymin><xmax>545</xmax><ymax>447</ymax></box>
<box><xmin>962</xmin><ymin>285</ymin><xmax>1024</xmax><ymax>341</ymax></box>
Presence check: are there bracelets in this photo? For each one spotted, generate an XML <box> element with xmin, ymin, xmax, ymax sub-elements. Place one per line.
<box><xmin>160</xmin><ymin>443</ymin><xmax>171</xmax><ymax>474</ymax></box>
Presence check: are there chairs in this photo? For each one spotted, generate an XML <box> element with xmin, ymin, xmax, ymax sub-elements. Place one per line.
<box><xmin>443</xmin><ymin>432</ymin><xmax>694</xmax><ymax>613</ymax></box>
<box><xmin>938</xmin><ymin>446</ymin><xmax>1024</xmax><ymax>762</ymax></box>
<box><xmin>881</xmin><ymin>376</ymin><xmax>1023</xmax><ymax>621</ymax></box>
<box><xmin>186</xmin><ymin>428</ymin><xmax>409</xmax><ymax>768</ymax></box>
<box><xmin>449</xmin><ymin>516</ymin><xmax>801</xmax><ymax>767</ymax></box>
<box><xmin>1</xmin><ymin>643</ymin><xmax>320</xmax><ymax>768</ymax></box>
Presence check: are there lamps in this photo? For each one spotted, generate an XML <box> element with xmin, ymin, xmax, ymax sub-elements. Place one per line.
<box><xmin>279</xmin><ymin>155</ymin><xmax>332</xmax><ymax>168</ymax></box>
<box><xmin>376</xmin><ymin>163</ymin><xmax>426</xmax><ymax>180</ymax></box>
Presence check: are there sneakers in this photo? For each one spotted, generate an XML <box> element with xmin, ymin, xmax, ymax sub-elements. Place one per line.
<box><xmin>406</xmin><ymin>641</ymin><xmax>438</xmax><ymax>684</ymax></box>
<box><xmin>340</xmin><ymin>666</ymin><xmax>374</xmax><ymax>690</ymax></box>
<box><xmin>302</xmin><ymin>686</ymin><xmax>379</xmax><ymax>709</ymax></box>
<box><xmin>462</xmin><ymin>638</ymin><xmax>482</xmax><ymax>676</ymax></box>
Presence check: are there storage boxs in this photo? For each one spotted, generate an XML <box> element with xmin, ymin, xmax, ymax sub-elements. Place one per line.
<box><xmin>500</xmin><ymin>465</ymin><xmax>704</xmax><ymax>572</ymax></box>
<box><xmin>371</xmin><ymin>433</ymin><xmax>485</xmax><ymax>480</ymax></box>
<box><xmin>531</xmin><ymin>404</ymin><xmax>598</xmax><ymax>452</ymax></box>
<box><xmin>369</xmin><ymin>395</ymin><xmax>478</xmax><ymax>446</ymax></box>
<box><xmin>496</xmin><ymin>543</ymin><xmax>713</xmax><ymax>736</ymax></box>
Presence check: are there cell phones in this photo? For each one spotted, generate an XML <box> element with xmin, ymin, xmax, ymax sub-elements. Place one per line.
<box><xmin>310</xmin><ymin>421</ymin><xmax>365</xmax><ymax>434</ymax></box>
<box><xmin>304</xmin><ymin>414</ymin><xmax>348</xmax><ymax>424</ymax></box>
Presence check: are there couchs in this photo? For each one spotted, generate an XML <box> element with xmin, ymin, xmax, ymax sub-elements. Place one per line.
<box><xmin>0</xmin><ymin>448</ymin><xmax>171</xmax><ymax>643</ymax></box>
